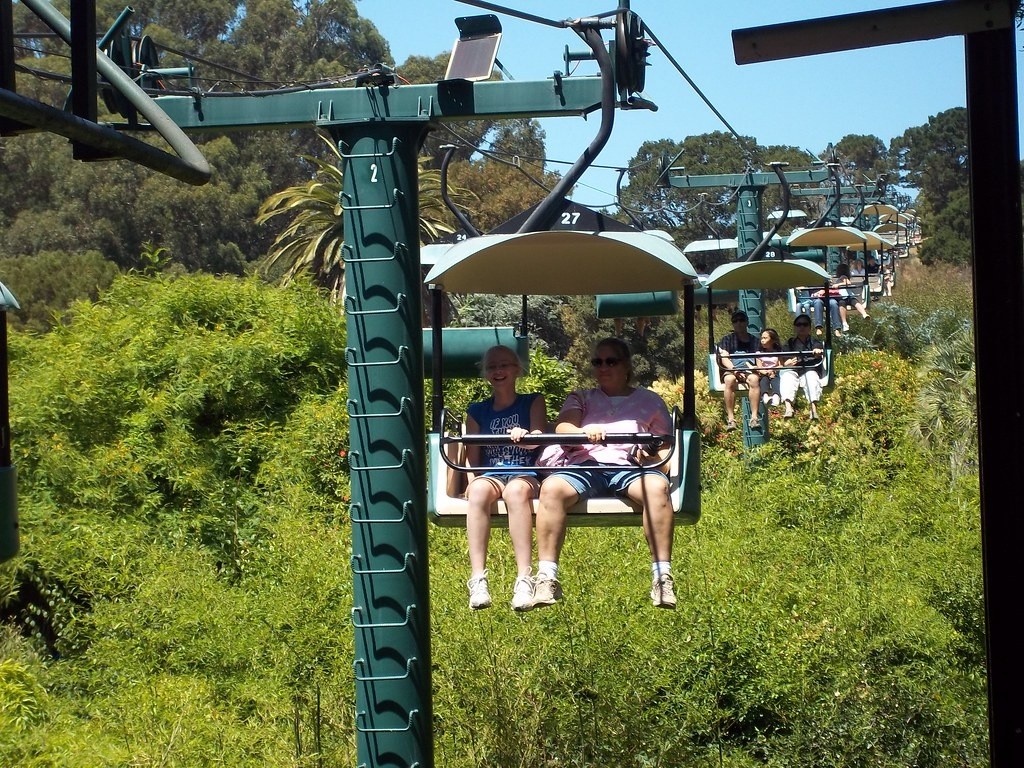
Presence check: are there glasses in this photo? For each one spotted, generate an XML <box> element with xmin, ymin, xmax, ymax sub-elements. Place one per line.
<box><xmin>731</xmin><ymin>316</ymin><xmax>746</xmax><ymax>323</ymax></box>
<box><xmin>591</xmin><ymin>357</ymin><xmax>623</xmax><ymax>367</ymax></box>
<box><xmin>795</xmin><ymin>322</ymin><xmax>811</xmax><ymax>327</ymax></box>
<box><xmin>486</xmin><ymin>360</ymin><xmax>518</xmax><ymax>371</ymax></box>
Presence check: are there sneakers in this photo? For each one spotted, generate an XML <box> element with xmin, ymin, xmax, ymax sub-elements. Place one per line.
<box><xmin>466</xmin><ymin>569</ymin><xmax>491</xmax><ymax>611</ymax></box>
<box><xmin>510</xmin><ymin>566</ymin><xmax>536</xmax><ymax>612</ymax></box>
<box><xmin>531</xmin><ymin>575</ymin><xmax>562</xmax><ymax>608</ymax></box>
<box><xmin>727</xmin><ymin>420</ymin><xmax>738</xmax><ymax>432</ymax></box>
<box><xmin>650</xmin><ymin>574</ymin><xmax>677</xmax><ymax>609</ymax></box>
<box><xmin>748</xmin><ymin>417</ymin><xmax>762</xmax><ymax>431</ymax></box>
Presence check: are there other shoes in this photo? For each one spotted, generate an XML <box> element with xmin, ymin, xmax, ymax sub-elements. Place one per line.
<box><xmin>816</xmin><ymin>327</ymin><xmax>823</xmax><ymax>335</ymax></box>
<box><xmin>771</xmin><ymin>394</ymin><xmax>779</xmax><ymax>407</ymax></box>
<box><xmin>783</xmin><ymin>408</ymin><xmax>795</xmax><ymax>418</ymax></box>
<box><xmin>888</xmin><ymin>291</ymin><xmax>892</xmax><ymax>296</ymax></box>
<box><xmin>763</xmin><ymin>397</ymin><xmax>773</xmax><ymax>406</ymax></box>
<box><xmin>862</xmin><ymin>314</ymin><xmax>870</xmax><ymax>320</ymax></box>
<box><xmin>882</xmin><ymin>291</ymin><xmax>888</xmax><ymax>296</ymax></box>
<box><xmin>843</xmin><ymin>322</ymin><xmax>849</xmax><ymax>332</ymax></box>
<box><xmin>835</xmin><ymin>329</ymin><xmax>841</xmax><ymax>337</ymax></box>
<box><xmin>809</xmin><ymin>413</ymin><xmax>819</xmax><ymax>421</ymax></box>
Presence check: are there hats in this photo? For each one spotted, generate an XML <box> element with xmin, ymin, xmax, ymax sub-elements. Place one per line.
<box><xmin>731</xmin><ymin>310</ymin><xmax>748</xmax><ymax>321</ymax></box>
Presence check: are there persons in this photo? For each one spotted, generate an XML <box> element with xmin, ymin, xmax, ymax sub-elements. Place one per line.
<box><xmin>533</xmin><ymin>335</ymin><xmax>679</xmax><ymax>608</ymax></box>
<box><xmin>613</xmin><ymin>316</ymin><xmax>648</xmax><ymax>343</ymax></box>
<box><xmin>755</xmin><ymin>328</ymin><xmax>781</xmax><ymax>406</ymax></box>
<box><xmin>779</xmin><ymin>313</ymin><xmax>824</xmax><ymax>420</ymax></box>
<box><xmin>463</xmin><ymin>341</ymin><xmax>546</xmax><ymax>613</ymax></box>
<box><xmin>794</xmin><ymin>249</ymin><xmax>893</xmax><ymax>337</ymax></box>
<box><xmin>717</xmin><ymin>310</ymin><xmax>763</xmax><ymax>431</ymax></box>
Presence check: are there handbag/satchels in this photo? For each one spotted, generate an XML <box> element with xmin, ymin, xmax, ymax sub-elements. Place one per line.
<box><xmin>730</xmin><ymin>351</ymin><xmax>755</xmax><ymax>373</ymax></box>
<box><xmin>791</xmin><ymin>354</ymin><xmax>822</xmax><ymax>366</ymax></box>
<box><xmin>535</xmin><ymin>390</ymin><xmax>585</xmax><ymax>474</ymax></box>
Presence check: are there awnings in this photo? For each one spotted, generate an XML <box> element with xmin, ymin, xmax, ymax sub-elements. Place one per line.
<box><xmin>702</xmin><ymin>261</ymin><xmax>834</xmax><ymax>289</ymax></box>
<box><xmin>681</xmin><ymin>231</ymin><xmax>783</xmax><ymax>254</ymax></box>
<box><xmin>421</xmin><ymin>226</ymin><xmax>699</xmax><ymax>296</ymax></box>
<box><xmin>767</xmin><ymin>203</ymin><xmax>922</xmax><ymax>252</ymax></box>
